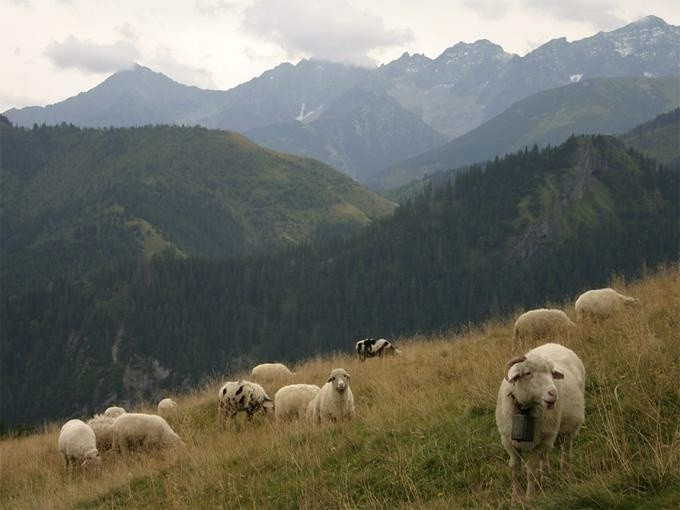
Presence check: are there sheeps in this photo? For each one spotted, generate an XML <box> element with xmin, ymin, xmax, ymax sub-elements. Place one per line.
<box><xmin>57</xmin><ymin>398</ymin><xmax>186</xmax><ymax>473</ymax></box>
<box><xmin>512</xmin><ymin>307</ymin><xmax>578</xmax><ymax>352</ymax></box>
<box><xmin>218</xmin><ymin>378</ymin><xmax>272</xmax><ymax>433</ymax></box>
<box><xmin>273</xmin><ymin>384</ymin><xmax>320</xmax><ymax>424</ymax></box>
<box><xmin>251</xmin><ymin>362</ymin><xmax>294</xmax><ymax>386</ymax></box>
<box><xmin>495</xmin><ymin>342</ymin><xmax>587</xmax><ymax>505</ymax></box>
<box><xmin>575</xmin><ymin>287</ymin><xmax>640</xmax><ymax>324</ymax></box>
<box><xmin>355</xmin><ymin>336</ymin><xmax>402</xmax><ymax>364</ymax></box>
<box><xmin>314</xmin><ymin>368</ymin><xmax>356</xmax><ymax>427</ymax></box>
